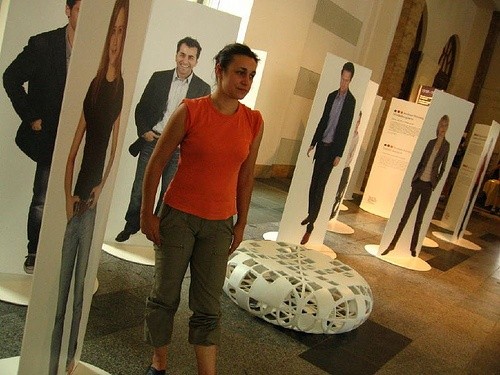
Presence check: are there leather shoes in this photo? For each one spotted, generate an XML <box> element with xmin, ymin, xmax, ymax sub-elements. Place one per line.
<box><xmin>300</xmin><ymin>215</ymin><xmax>310</xmax><ymax>225</ymax></box>
<box><xmin>300</xmin><ymin>232</ymin><xmax>311</xmax><ymax>245</ymax></box>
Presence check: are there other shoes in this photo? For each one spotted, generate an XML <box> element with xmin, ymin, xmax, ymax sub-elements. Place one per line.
<box><xmin>381</xmin><ymin>245</ymin><xmax>394</xmax><ymax>255</ymax></box>
<box><xmin>146</xmin><ymin>362</ymin><xmax>167</xmax><ymax>375</ymax></box>
<box><xmin>410</xmin><ymin>249</ymin><xmax>417</xmax><ymax>257</ymax></box>
<box><xmin>115</xmin><ymin>223</ymin><xmax>140</xmax><ymax>242</ymax></box>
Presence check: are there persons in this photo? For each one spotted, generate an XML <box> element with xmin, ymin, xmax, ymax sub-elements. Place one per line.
<box><xmin>479</xmin><ymin>161</ymin><xmax>500</xmax><ymax>214</ymax></box>
<box><xmin>48</xmin><ymin>0</ymin><xmax>130</xmax><ymax>375</ymax></box>
<box><xmin>299</xmin><ymin>62</ymin><xmax>357</xmax><ymax>245</ymax></box>
<box><xmin>140</xmin><ymin>44</ymin><xmax>264</xmax><ymax>375</ymax></box>
<box><xmin>452</xmin><ymin>137</ymin><xmax>466</xmax><ymax>168</ymax></box>
<box><xmin>329</xmin><ymin>111</ymin><xmax>364</xmax><ymax>221</ymax></box>
<box><xmin>115</xmin><ymin>37</ymin><xmax>211</xmax><ymax>243</ymax></box>
<box><xmin>3</xmin><ymin>1</ymin><xmax>82</xmax><ymax>274</ymax></box>
<box><xmin>381</xmin><ymin>115</ymin><xmax>450</xmax><ymax>257</ymax></box>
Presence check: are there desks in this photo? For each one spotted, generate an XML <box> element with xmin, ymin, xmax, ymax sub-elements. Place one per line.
<box><xmin>483</xmin><ymin>180</ymin><xmax>500</xmax><ymax>214</ymax></box>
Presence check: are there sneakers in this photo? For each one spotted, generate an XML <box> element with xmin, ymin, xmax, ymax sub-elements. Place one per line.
<box><xmin>23</xmin><ymin>256</ymin><xmax>36</xmax><ymax>274</ymax></box>
